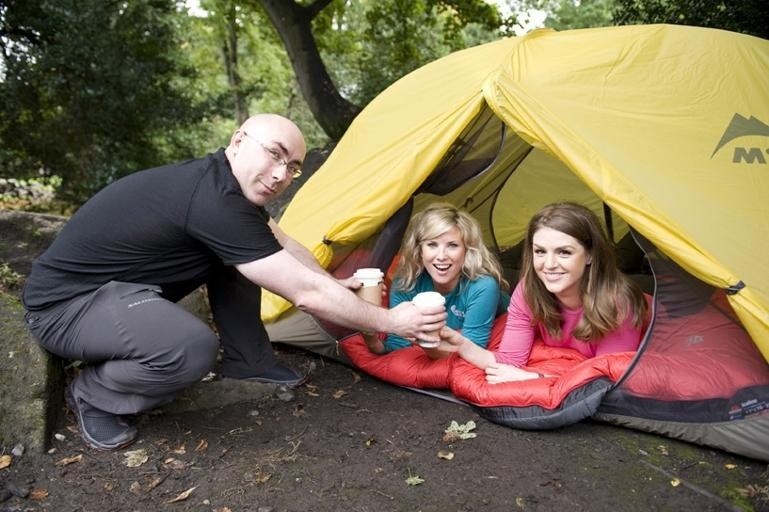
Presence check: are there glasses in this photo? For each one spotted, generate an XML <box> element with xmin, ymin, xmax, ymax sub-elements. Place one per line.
<box><xmin>241</xmin><ymin>130</ymin><xmax>302</xmax><ymax>179</ymax></box>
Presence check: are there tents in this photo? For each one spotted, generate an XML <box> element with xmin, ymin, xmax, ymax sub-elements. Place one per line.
<box><xmin>261</xmin><ymin>23</ymin><xmax>769</xmax><ymax>464</ymax></box>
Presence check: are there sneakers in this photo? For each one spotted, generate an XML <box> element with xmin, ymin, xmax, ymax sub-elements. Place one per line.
<box><xmin>224</xmin><ymin>359</ymin><xmax>309</xmax><ymax>386</ymax></box>
<box><xmin>64</xmin><ymin>377</ymin><xmax>139</xmax><ymax>452</ymax></box>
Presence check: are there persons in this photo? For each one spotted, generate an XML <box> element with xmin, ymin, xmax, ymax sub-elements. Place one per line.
<box><xmin>22</xmin><ymin>114</ymin><xmax>448</xmax><ymax>451</ymax></box>
<box><xmin>351</xmin><ymin>202</ymin><xmax>512</xmax><ymax>359</ymax></box>
<box><xmin>434</xmin><ymin>202</ymin><xmax>716</xmax><ymax>383</ymax></box>
